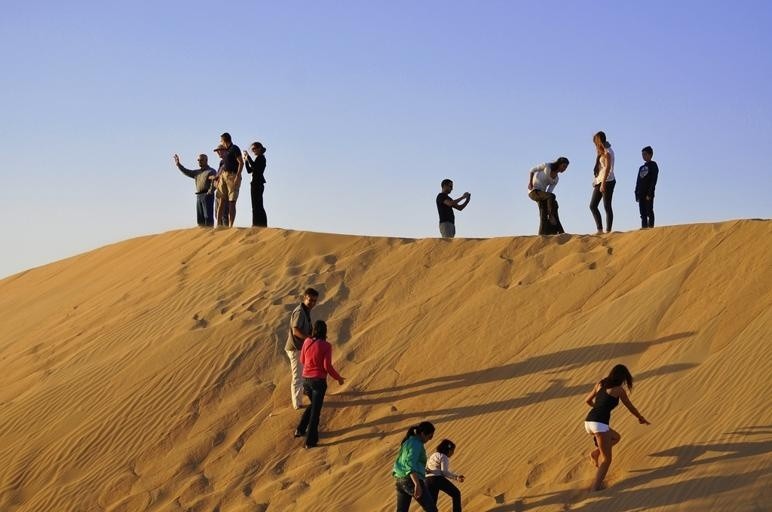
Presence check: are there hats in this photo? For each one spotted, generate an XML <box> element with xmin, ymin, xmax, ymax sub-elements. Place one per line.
<box><xmin>213</xmin><ymin>144</ymin><xmax>226</xmax><ymax>151</ymax></box>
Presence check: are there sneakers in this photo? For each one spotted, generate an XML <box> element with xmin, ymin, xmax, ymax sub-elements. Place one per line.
<box><xmin>294</xmin><ymin>430</ymin><xmax>306</xmax><ymax>436</ymax></box>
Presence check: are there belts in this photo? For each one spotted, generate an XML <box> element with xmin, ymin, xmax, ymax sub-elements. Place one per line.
<box><xmin>196</xmin><ymin>192</ymin><xmax>206</xmax><ymax>194</ymax></box>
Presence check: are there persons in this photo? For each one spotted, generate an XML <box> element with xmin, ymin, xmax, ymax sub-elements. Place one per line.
<box><xmin>583</xmin><ymin>363</ymin><xmax>651</xmax><ymax>493</ymax></box>
<box><xmin>634</xmin><ymin>145</ymin><xmax>659</xmax><ymax>228</ymax></box>
<box><xmin>588</xmin><ymin>130</ymin><xmax>617</xmax><ymax>233</ymax></box>
<box><xmin>527</xmin><ymin>156</ymin><xmax>570</xmax><ymax>235</ymax></box>
<box><xmin>293</xmin><ymin>319</ymin><xmax>346</xmax><ymax>450</ymax></box>
<box><xmin>390</xmin><ymin>420</ymin><xmax>440</xmax><ymax>512</ymax></box>
<box><xmin>425</xmin><ymin>438</ymin><xmax>465</xmax><ymax>512</ymax></box>
<box><xmin>173</xmin><ymin>132</ymin><xmax>268</xmax><ymax>227</ymax></box>
<box><xmin>283</xmin><ymin>287</ymin><xmax>320</xmax><ymax>411</ymax></box>
<box><xmin>435</xmin><ymin>178</ymin><xmax>471</xmax><ymax>237</ymax></box>
